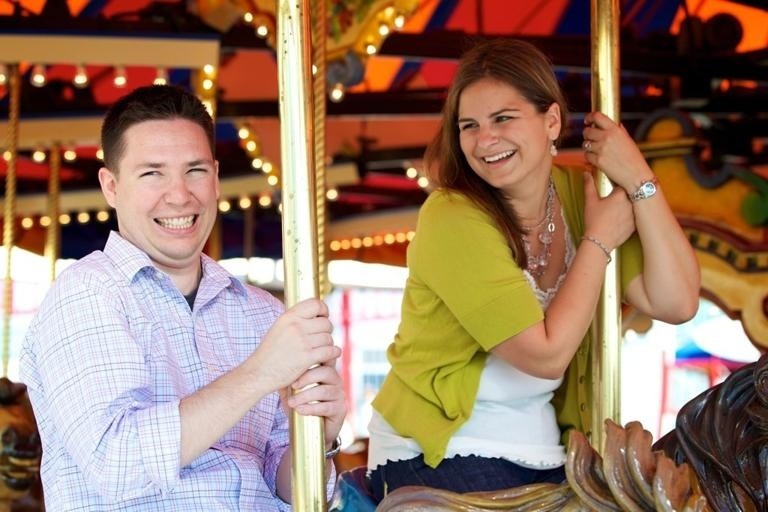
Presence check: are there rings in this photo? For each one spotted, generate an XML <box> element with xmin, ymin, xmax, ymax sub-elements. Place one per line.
<box><xmin>583</xmin><ymin>140</ymin><xmax>593</xmax><ymax>152</ymax></box>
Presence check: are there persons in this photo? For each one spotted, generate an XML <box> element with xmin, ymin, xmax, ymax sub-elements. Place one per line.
<box><xmin>19</xmin><ymin>84</ymin><xmax>352</xmax><ymax>510</ymax></box>
<box><xmin>361</xmin><ymin>40</ymin><xmax>703</xmax><ymax>504</ymax></box>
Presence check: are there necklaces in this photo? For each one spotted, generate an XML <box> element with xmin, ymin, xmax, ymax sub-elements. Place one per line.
<box><xmin>503</xmin><ymin>176</ymin><xmax>565</xmax><ymax>290</ymax></box>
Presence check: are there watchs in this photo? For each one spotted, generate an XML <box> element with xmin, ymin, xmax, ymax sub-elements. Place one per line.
<box><xmin>620</xmin><ymin>176</ymin><xmax>662</xmax><ymax>206</ymax></box>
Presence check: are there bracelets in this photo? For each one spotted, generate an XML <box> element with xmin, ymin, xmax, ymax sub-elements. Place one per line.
<box><xmin>315</xmin><ymin>437</ymin><xmax>345</xmax><ymax>456</ymax></box>
<box><xmin>580</xmin><ymin>234</ymin><xmax>615</xmax><ymax>264</ymax></box>
